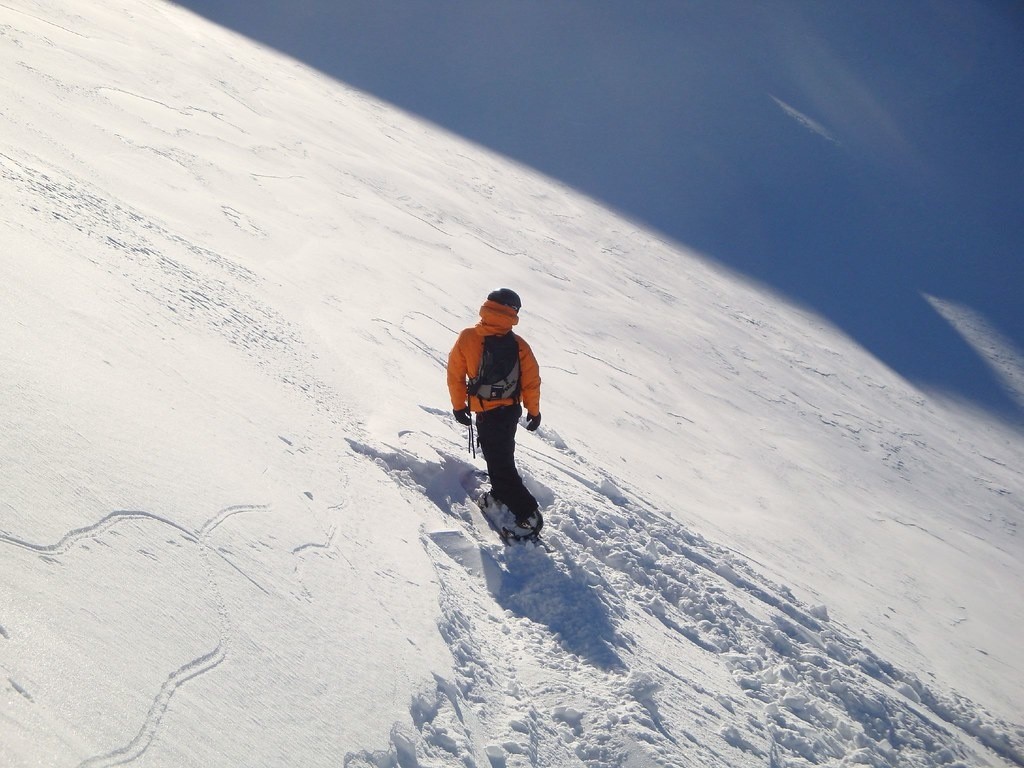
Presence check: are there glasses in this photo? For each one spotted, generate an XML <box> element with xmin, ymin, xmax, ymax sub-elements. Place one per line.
<box><xmin>511</xmin><ymin>306</ymin><xmax>520</xmax><ymax>310</ymax></box>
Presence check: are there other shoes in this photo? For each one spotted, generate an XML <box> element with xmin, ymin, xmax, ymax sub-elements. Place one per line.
<box><xmin>478</xmin><ymin>491</ymin><xmax>505</xmax><ymax>507</ymax></box>
<box><xmin>502</xmin><ymin>509</ymin><xmax>543</xmax><ymax>539</ymax></box>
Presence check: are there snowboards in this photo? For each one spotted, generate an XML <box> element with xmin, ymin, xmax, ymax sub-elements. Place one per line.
<box><xmin>461</xmin><ymin>466</ymin><xmax>543</xmax><ymax>545</ymax></box>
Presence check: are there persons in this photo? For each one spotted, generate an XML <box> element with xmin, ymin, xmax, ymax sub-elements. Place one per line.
<box><xmin>447</xmin><ymin>288</ymin><xmax>544</xmax><ymax>537</ymax></box>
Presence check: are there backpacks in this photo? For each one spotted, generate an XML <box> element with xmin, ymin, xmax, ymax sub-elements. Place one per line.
<box><xmin>466</xmin><ymin>330</ymin><xmax>521</xmax><ymax>402</ymax></box>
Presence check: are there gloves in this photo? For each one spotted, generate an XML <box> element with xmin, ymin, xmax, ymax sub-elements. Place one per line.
<box><xmin>453</xmin><ymin>406</ymin><xmax>472</xmax><ymax>426</ymax></box>
<box><xmin>525</xmin><ymin>412</ymin><xmax>542</xmax><ymax>431</ymax></box>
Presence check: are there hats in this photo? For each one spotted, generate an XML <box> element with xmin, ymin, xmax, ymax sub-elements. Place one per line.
<box><xmin>489</xmin><ymin>288</ymin><xmax>521</xmax><ymax>309</ymax></box>
